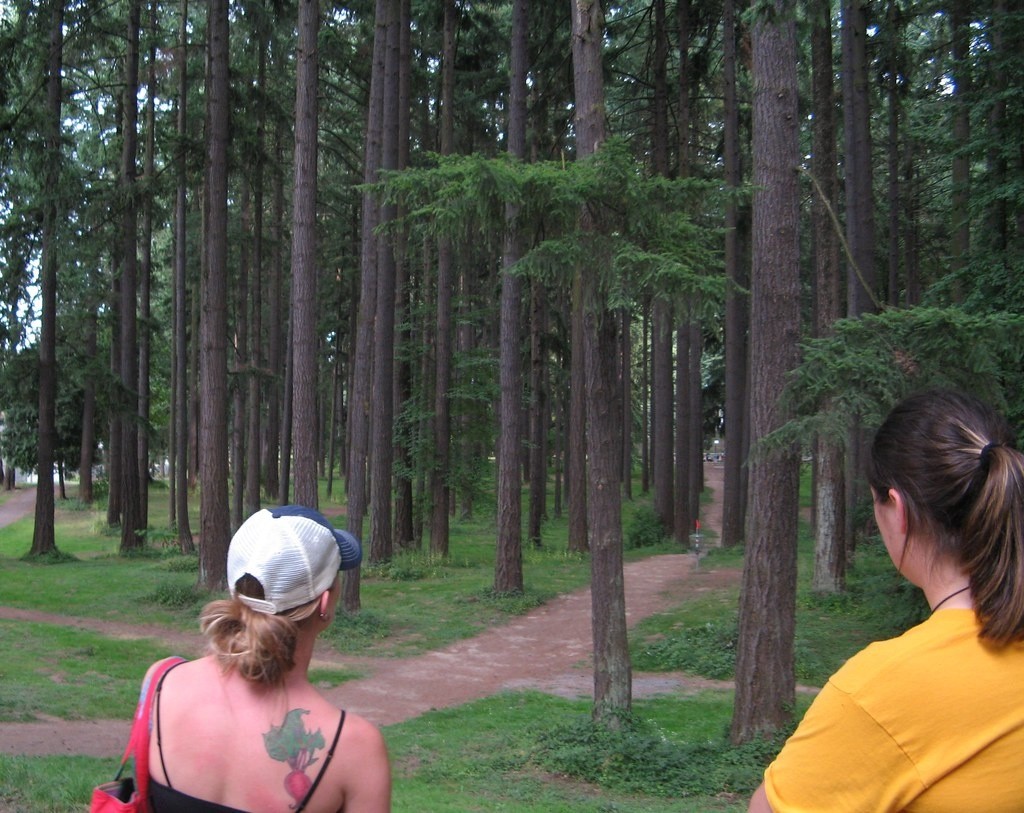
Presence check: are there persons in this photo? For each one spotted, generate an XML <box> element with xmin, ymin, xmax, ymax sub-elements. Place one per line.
<box><xmin>706</xmin><ymin>451</ymin><xmax>710</xmax><ymax>463</ymax></box>
<box><xmin>133</xmin><ymin>505</ymin><xmax>392</xmax><ymax>813</ymax></box>
<box><xmin>746</xmin><ymin>389</ymin><xmax>1024</xmax><ymax>813</ymax></box>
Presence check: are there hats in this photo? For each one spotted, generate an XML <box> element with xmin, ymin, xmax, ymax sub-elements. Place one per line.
<box><xmin>227</xmin><ymin>503</ymin><xmax>363</xmax><ymax>614</ymax></box>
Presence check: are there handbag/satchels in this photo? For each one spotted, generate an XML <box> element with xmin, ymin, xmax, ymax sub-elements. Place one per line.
<box><xmin>90</xmin><ymin>656</ymin><xmax>189</xmax><ymax>813</ymax></box>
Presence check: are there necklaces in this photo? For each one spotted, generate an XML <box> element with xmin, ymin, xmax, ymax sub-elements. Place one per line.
<box><xmin>931</xmin><ymin>585</ymin><xmax>969</xmax><ymax>615</ymax></box>
